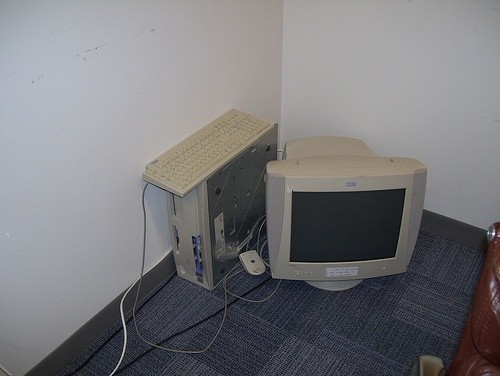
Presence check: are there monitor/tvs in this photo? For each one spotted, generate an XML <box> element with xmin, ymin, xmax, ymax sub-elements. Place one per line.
<box><xmin>266</xmin><ymin>135</ymin><xmax>428</xmax><ymax>281</ymax></box>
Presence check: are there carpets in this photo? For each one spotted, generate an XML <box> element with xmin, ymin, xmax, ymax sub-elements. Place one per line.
<box><xmin>61</xmin><ymin>229</ymin><xmax>488</xmax><ymax>376</ymax></box>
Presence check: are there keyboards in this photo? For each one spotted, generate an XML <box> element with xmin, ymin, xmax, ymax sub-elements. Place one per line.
<box><xmin>141</xmin><ymin>107</ymin><xmax>276</xmax><ymax>199</ymax></box>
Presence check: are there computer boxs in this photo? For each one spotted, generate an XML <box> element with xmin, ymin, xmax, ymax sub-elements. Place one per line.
<box><xmin>165</xmin><ymin>123</ymin><xmax>279</xmax><ymax>292</ymax></box>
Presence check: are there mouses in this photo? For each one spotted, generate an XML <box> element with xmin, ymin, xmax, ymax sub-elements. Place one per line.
<box><xmin>237</xmin><ymin>248</ymin><xmax>266</xmax><ymax>276</ymax></box>
